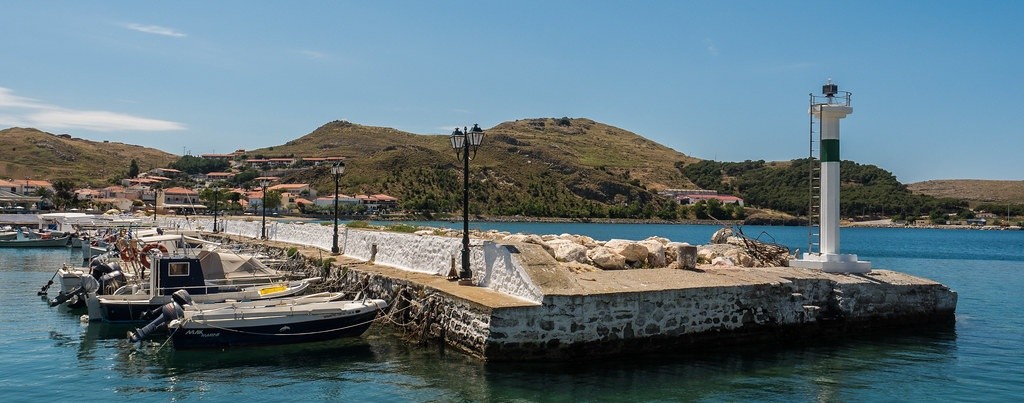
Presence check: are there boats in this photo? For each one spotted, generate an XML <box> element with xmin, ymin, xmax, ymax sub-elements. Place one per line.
<box><xmin>0</xmin><ymin>230</ymin><xmax>70</xmax><ymax>247</ymax></box>
<box><xmin>97</xmin><ymin>289</ymin><xmax>344</xmax><ymax>326</ymax></box>
<box><xmin>149</xmin><ymin>299</ymin><xmax>390</xmax><ymax>345</ymax></box>
<box><xmin>58</xmin><ymin>221</ymin><xmax>323</xmax><ymax>327</ymax></box>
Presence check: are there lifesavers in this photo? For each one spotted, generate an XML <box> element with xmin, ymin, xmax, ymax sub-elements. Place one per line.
<box><xmin>141</xmin><ymin>243</ymin><xmax>169</xmax><ymax>268</ymax></box>
<box><xmin>121</xmin><ymin>245</ymin><xmax>137</xmax><ymax>262</ymax></box>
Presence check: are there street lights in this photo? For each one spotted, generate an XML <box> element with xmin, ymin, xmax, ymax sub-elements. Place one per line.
<box><xmin>449</xmin><ymin>123</ymin><xmax>488</xmax><ymax>284</ymax></box>
<box><xmin>213</xmin><ymin>186</ymin><xmax>219</xmax><ymax>232</ymax></box>
<box><xmin>259</xmin><ymin>177</ymin><xmax>272</xmax><ymax>240</ymax></box>
<box><xmin>330</xmin><ymin>161</ymin><xmax>346</xmax><ymax>253</ymax></box>
<box><xmin>149</xmin><ymin>187</ymin><xmax>160</xmax><ymax>222</ymax></box>
